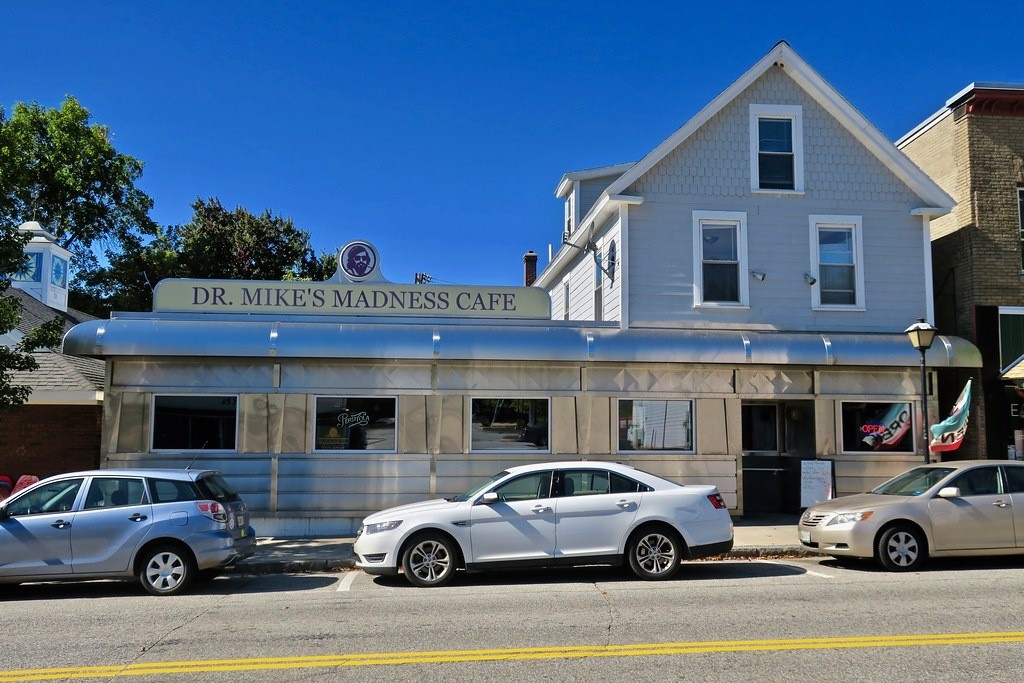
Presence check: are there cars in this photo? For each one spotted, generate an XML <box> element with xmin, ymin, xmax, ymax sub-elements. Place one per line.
<box><xmin>797</xmin><ymin>459</ymin><xmax>1024</xmax><ymax>573</ymax></box>
<box><xmin>352</xmin><ymin>462</ymin><xmax>735</xmax><ymax>588</ymax></box>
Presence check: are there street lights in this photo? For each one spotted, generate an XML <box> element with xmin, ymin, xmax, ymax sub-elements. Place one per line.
<box><xmin>903</xmin><ymin>316</ymin><xmax>939</xmax><ymax>489</ymax></box>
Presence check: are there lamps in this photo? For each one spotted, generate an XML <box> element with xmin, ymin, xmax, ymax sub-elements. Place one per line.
<box><xmin>803</xmin><ymin>273</ymin><xmax>817</xmax><ymax>286</ymax></box>
<box><xmin>562</xmin><ymin>230</ymin><xmax>588</xmax><ymax>254</ymax></box>
<box><xmin>750</xmin><ymin>270</ymin><xmax>766</xmax><ymax>281</ymax></box>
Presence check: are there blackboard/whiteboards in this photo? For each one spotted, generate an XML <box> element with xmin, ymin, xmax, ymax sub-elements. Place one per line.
<box><xmin>800</xmin><ymin>459</ymin><xmax>836</xmax><ymax>508</ymax></box>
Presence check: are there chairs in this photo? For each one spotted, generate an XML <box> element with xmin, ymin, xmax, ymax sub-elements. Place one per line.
<box><xmin>93</xmin><ymin>487</ymin><xmax>105</xmax><ymax>506</ymax></box>
<box><xmin>545</xmin><ymin>477</ymin><xmax>575</xmax><ymax>497</ymax></box>
<box><xmin>110</xmin><ymin>490</ymin><xmax>127</xmax><ymax>505</ymax></box>
<box><xmin>959</xmin><ymin>475</ymin><xmax>977</xmax><ymax>496</ymax></box>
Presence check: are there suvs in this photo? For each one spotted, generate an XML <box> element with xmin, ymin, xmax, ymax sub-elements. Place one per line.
<box><xmin>0</xmin><ymin>469</ymin><xmax>257</xmax><ymax>597</ymax></box>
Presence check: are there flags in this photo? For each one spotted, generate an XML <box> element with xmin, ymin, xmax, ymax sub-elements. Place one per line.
<box><xmin>860</xmin><ymin>402</ymin><xmax>911</xmax><ymax>450</ymax></box>
<box><xmin>930</xmin><ymin>379</ymin><xmax>973</xmax><ymax>452</ymax></box>
